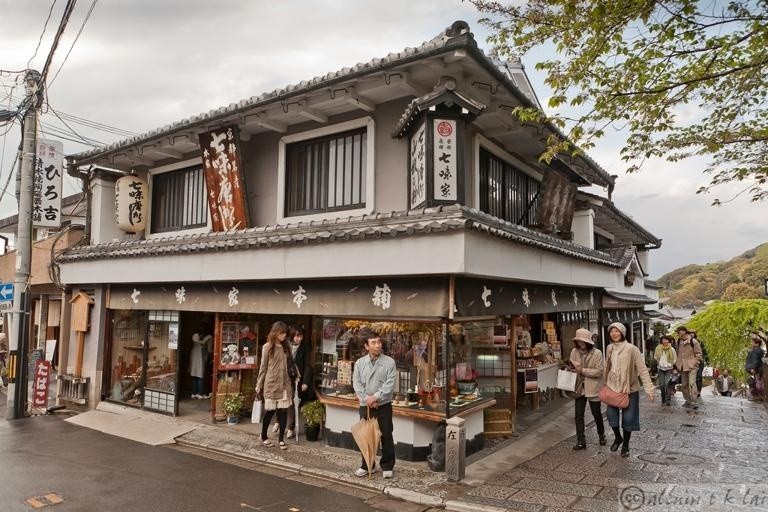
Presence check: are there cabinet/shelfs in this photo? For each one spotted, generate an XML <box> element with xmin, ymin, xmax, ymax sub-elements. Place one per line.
<box><xmin>216</xmin><ymin>320</ymin><xmax>259</xmax><ymax>370</ymax></box>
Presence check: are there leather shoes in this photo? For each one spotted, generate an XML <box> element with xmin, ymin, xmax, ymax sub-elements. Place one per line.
<box><xmin>598</xmin><ymin>433</ymin><xmax>606</xmax><ymax>445</ymax></box>
<box><xmin>621</xmin><ymin>446</ymin><xmax>629</xmax><ymax>457</ymax></box>
<box><xmin>571</xmin><ymin>440</ymin><xmax>587</xmax><ymax>450</ymax></box>
<box><xmin>609</xmin><ymin>435</ymin><xmax>623</xmax><ymax>451</ymax></box>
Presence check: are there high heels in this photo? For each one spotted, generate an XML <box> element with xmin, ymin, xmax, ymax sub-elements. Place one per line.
<box><xmin>258</xmin><ymin>438</ymin><xmax>275</xmax><ymax>447</ymax></box>
<box><xmin>278</xmin><ymin>440</ymin><xmax>287</xmax><ymax>450</ymax></box>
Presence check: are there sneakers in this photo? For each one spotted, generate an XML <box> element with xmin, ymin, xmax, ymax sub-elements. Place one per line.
<box><xmin>662</xmin><ymin>398</ymin><xmax>666</xmax><ymax>403</ymax></box>
<box><xmin>287</xmin><ymin>428</ymin><xmax>295</xmax><ymax>438</ymax></box>
<box><xmin>666</xmin><ymin>400</ymin><xmax>670</xmax><ymax>405</ymax></box>
<box><xmin>681</xmin><ymin>400</ymin><xmax>689</xmax><ymax>408</ymax></box>
<box><xmin>272</xmin><ymin>422</ymin><xmax>280</xmax><ymax>433</ymax></box>
<box><xmin>198</xmin><ymin>394</ymin><xmax>210</xmax><ymax>399</ymax></box>
<box><xmin>692</xmin><ymin>400</ymin><xmax>699</xmax><ymax>406</ymax></box>
<box><xmin>356</xmin><ymin>466</ymin><xmax>377</xmax><ymax>477</ymax></box>
<box><xmin>191</xmin><ymin>393</ymin><xmax>199</xmax><ymax>399</ymax></box>
<box><xmin>382</xmin><ymin>471</ymin><xmax>394</xmax><ymax>477</ymax></box>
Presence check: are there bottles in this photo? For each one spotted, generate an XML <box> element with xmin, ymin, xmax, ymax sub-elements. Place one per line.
<box><xmin>404</xmin><ymin>394</ymin><xmax>410</xmax><ymax>405</ymax></box>
<box><xmin>419</xmin><ymin>399</ymin><xmax>423</xmax><ymax>408</ymax></box>
<box><xmin>394</xmin><ymin>395</ymin><xmax>400</xmax><ymax>404</ymax></box>
<box><xmin>323</xmin><ymin>361</ymin><xmax>327</xmax><ymax>373</ymax></box>
<box><xmin>326</xmin><ymin>363</ymin><xmax>331</xmax><ymax>374</ymax></box>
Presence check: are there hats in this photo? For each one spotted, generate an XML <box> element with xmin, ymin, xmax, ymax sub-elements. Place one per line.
<box><xmin>607</xmin><ymin>322</ymin><xmax>626</xmax><ymax>337</ymax></box>
<box><xmin>674</xmin><ymin>325</ymin><xmax>687</xmax><ymax>333</ymax></box>
<box><xmin>570</xmin><ymin>327</ymin><xmax>595</xmax><ymax>344</ymax></box>
<box><xmin>688</xmin><ymin>329</ymin><xmax>696</xmax><ymax>333</ymax></box>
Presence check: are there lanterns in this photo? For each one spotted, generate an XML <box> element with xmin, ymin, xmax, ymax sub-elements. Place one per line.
<box><xmin>114</xmin><ymin>172</ymin><xmax>148</xmax><ymax>240</ymax></box>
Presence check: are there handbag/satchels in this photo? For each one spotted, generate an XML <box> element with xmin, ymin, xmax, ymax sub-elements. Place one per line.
<box><xmin>702</xmin><ymin>366</ymin><xmax>714</xmax><ymax>377</ymax></box>
<box><xmin>747</xmin><ymin>375</ymin><xmax>756</xmax><ymax>386</ymax></box>
<box><xmin>250</xmin><ymin>398</ymin><xmax>262</xmax><ymax>423</ymax></box>
<box><xmin>749</xmin><ymin>380</ymin><xmax>763</xmax><ymax>396</ymax></box>
<box><xmin>555</xmin><ymin>370</ymin><xmax>577</xmax><ymax>391</ymax></box>
<box><xmin>598</xmin><ymin>384</ymin><xmax>631</xmax><ymax>409</ymax></box>
<box><xmin>566</xmin><ymin>375</ymin><xmax>583</xmax><ymax>397</ymax></box>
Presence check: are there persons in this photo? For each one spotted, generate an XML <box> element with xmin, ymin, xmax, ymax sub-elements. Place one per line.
<box><xmin>0</xmin><ymin>333</ymin><xmax>8</xmax><ymax>387</ymax></box>
<box><xmin>351</xmin><ymin>333</ymin><xmax>397</xmax><ymax>479</ymax></box>
<box><xmin>189</xmin><ymin>332</ymin><xmax>212</xmax><ymax>400</ymax></box>
<box><xmin>604</xmin><ymin>322</ymin><xmax>655</xmax><ymax>458</ymax></box>
<box><xmin>569</xmin><ymin>328</ymin><xmax>607</xmax><ymax>450</ymax></box>
<box><xmin>255</xmin><ymin>320</ymin><xmax>302</xmax><ymax>450</ymax></box>
<box><xmin>653</xmin><ymin>326</ymin><xmax>710</xmax><ymax>409</ymax></box>
<box><xmin>272</xmin><ymin>324</ymin><xmax>313</xmax><ymax>440</ymax></box>
<box><xmin>450</xmin><ymin>326</ymin><xmax>473</xmax><ymax>382</ymax></box>
<box><xmin>717</xmin><ymin>369</ymin><xmax>734</xmax><ymax>397</ymax></box>
<box><xmin>745</xmin><ymin>337</ymin><xmax>764</xmax><ymax>401</ymax></box>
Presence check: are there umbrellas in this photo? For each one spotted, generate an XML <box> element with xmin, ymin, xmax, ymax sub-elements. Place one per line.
<box><xmin>292</xmin><ymin>380</ymin><xmax>301</xmax><ymax>442</ymax></box>
<box><xmin>352</xmin><ymin>404</ymin><xmax>383</xmax><ymax>479</ymax></box>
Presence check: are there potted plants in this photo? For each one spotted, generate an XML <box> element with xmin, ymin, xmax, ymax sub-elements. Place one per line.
<box><xmin>301</xmin><ymin>400</ymin><xmax>325</xmax><ymax>441</ymax></box>
<box><xmin>224</xmin><ymin>392</ymin><xmax>248</xmax><ymax>425</ymax></box>
